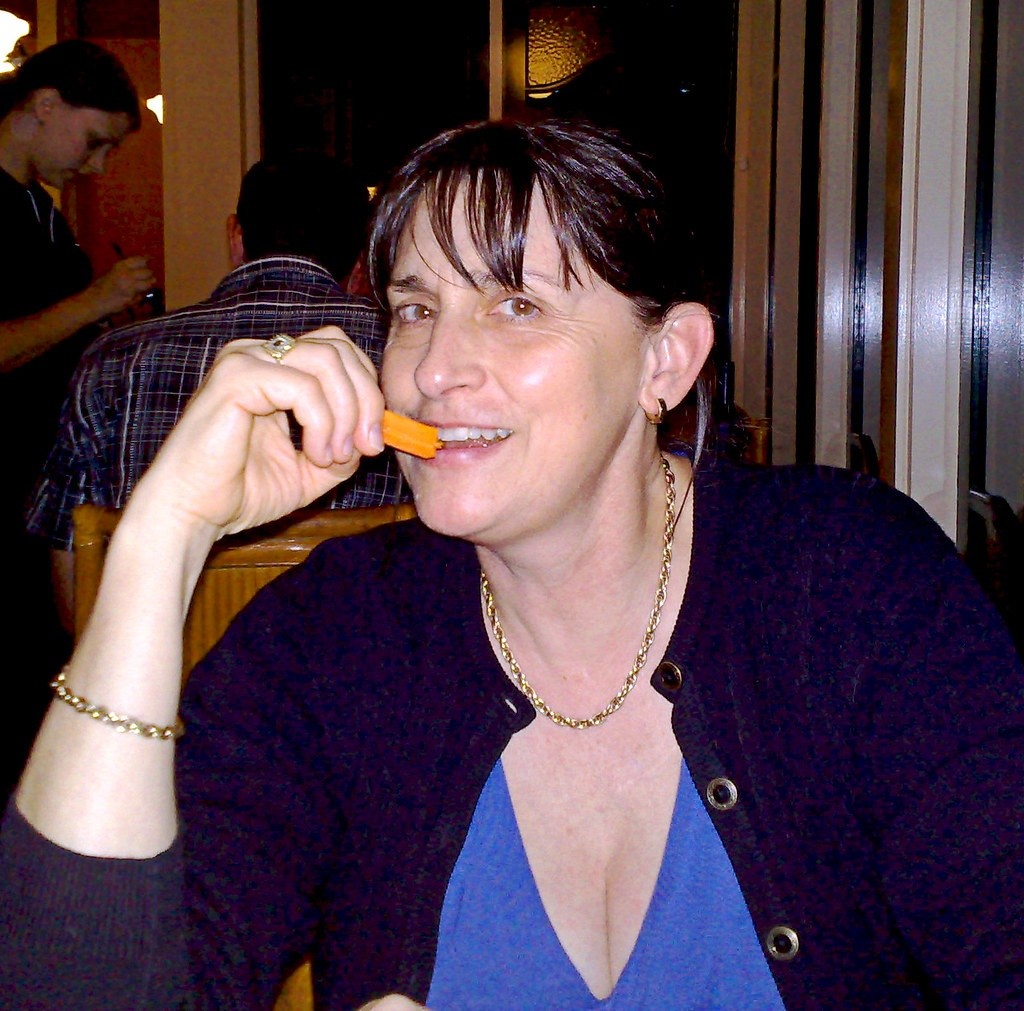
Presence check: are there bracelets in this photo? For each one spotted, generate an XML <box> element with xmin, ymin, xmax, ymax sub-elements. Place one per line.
<box><xmin>51</xmin><ymin>662</ymin><xmax>184</xmax><ymax>740</ymax></box>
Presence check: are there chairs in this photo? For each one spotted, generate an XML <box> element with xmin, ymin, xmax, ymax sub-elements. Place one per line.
<box><xmin>73</xmin><ymin>504</ymin><xmax>417</xmax><ymax>1011</ymax></box>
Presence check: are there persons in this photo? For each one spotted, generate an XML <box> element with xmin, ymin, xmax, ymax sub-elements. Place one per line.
<box><xmin>24</xmin><ymin>150</ymin><xmax>412</xmax><ymax>635</ymax></box>
<box><xmin>0</xmin><ymin>36</ymin><xmax>156</xmax><ymax>826</ymax></box>
<box><xmin>0</xmin><ymin>113</ymin><xmax>1024</xmax><ymax>1011</ymax></box>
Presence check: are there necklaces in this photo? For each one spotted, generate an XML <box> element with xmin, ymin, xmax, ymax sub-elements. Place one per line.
<box><xmin>481</xmin><ymin>449</ymin><xmax>676</xmax><ymax>729</ymax></box>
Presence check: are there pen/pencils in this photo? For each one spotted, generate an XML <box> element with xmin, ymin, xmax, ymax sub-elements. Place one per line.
<box><xmin>110</xmin><ymin>243</ymin><xmax>125</xmax><ymax>260</ymax></box>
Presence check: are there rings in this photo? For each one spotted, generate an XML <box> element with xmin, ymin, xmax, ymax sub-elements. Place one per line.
<box><xmin>261</xmin><ymin>332</ymin><xmax>294</xmax><ymax>365</ymax></box>
